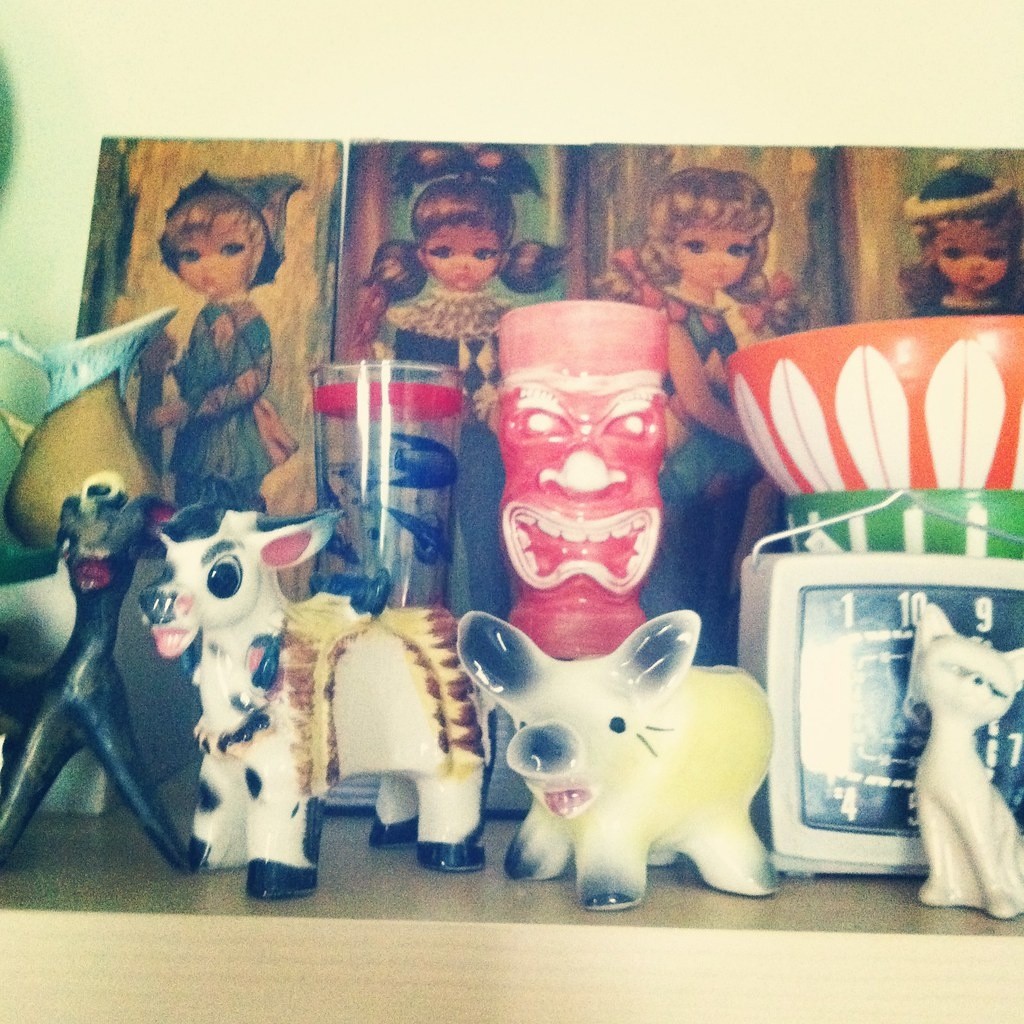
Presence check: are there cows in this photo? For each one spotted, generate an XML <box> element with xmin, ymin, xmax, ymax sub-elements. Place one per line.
<box><xmin>129</xmin><ymin>500</ymin><xmax>493</xmax><ymax>902</ymax></box>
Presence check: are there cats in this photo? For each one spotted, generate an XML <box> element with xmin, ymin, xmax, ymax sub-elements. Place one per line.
<box><xmin>907</xmin><ymin>598</ymin><xmax>1023</xmax><ymax>922</ymax></box>
